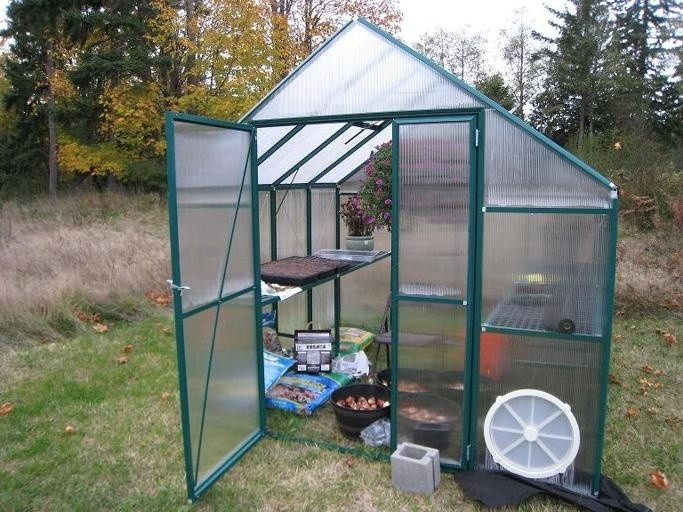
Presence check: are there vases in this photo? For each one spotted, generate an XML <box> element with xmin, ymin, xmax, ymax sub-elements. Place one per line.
<box><xmin>347</xmin><ymin>236</ymin><xmax>373</xmax><ymax>252</ymax></box>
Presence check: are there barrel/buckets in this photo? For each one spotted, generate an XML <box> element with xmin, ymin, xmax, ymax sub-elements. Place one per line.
<box><xmin>331</xmin><ymin>370</ymin><xmax>490</xmax><ymax>450</ymax></box>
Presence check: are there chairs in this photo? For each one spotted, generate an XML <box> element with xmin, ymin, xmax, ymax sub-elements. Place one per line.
<box><xmin>372</xmin><ymin>283</ymin><xmax>461</xmax><ymax>384</ymax></box>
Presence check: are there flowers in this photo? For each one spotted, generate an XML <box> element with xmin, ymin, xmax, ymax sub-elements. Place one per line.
<box><xmin>357</xmin><ymin>137</ymin><xmax>469</xmax><ymax>233</ymax></box>
<box><xmin>339</xmin><ymin>197</ymin><xmax>375</xmax><ymax>236</ymax></box>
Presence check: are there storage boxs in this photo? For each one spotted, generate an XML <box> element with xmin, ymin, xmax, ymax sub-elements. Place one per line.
<box><xmin>294</xmin><ymin>330</ymin><xmax>332</xmax><ymax>372</ymax></box>
